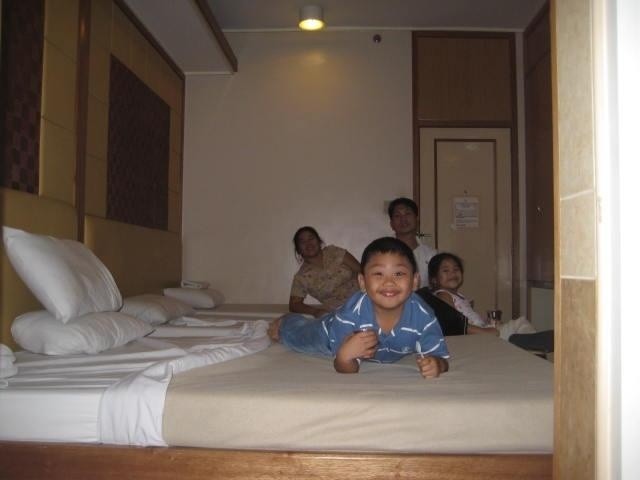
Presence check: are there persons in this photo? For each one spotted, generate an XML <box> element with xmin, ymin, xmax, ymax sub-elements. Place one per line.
<box><xmin>288</xmin><ymin>226</ymin><xmax>363</xmax><ymax>321</ymax></box>
<box><xmin>388</xmin><ymin>196</ymin><xmax>441</xmax><ymax>291</ymax></box>
<box><xmin>415</xmin><ymin>252</ymin><xmax>546</xmax><ymax>361</ymax></box>
<box><xmin>267</xmin><ymin>237</ymin><xmax>450</xmax><ymax>379</ymax></box>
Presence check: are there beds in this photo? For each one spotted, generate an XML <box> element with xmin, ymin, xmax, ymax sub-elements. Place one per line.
<box><xmin>0</xmin><ymin>191</ymin><xmax>554</xmax><ymax>477</ymax></box>
<box><xmin>85</xmin><ymin>215</ymin><xmax>498</xmax><ymax>335</ymax></box>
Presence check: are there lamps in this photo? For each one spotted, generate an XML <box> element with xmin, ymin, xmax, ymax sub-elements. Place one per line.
<box><xmin>297</xmin><ymin>2</ymin><xmax>323</xmax><ymax>31</ymax></box>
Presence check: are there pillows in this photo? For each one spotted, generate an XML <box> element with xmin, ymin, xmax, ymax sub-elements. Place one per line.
<box><xmin>8</xmin><ymin>307</ymin><xmax>156</xmax><ymax>355</ymax></box>
<box><xmin>120</xmin><ymin>294</ymin><xmax>195</xmax><ymax>322</ymax></box>
<box><xmin>3</xmin><ymin>227</ymin><xmax>121</xmax><ymax>323</ymax></box>
<box><xmin>165</xmin><ymin>287</ymin><xmax>224</xmax><ymax>307</ymax></box>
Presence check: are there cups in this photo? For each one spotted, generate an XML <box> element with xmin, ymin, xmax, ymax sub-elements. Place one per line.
<box><xmin>354</xmin><ymin>328</ymin><xmax>375</xmax><ymax>360</ymax></box>
<box><xmin>488</xmin><ymin>310</ymin><xmax>503</xmax><ymax>321</ymax></box>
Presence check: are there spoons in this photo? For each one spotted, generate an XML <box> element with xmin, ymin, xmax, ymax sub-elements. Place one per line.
<box><xmin>414</xmin><ymin>341</ymin><xmax>425</xmax><ymax>360</ymax></box>
